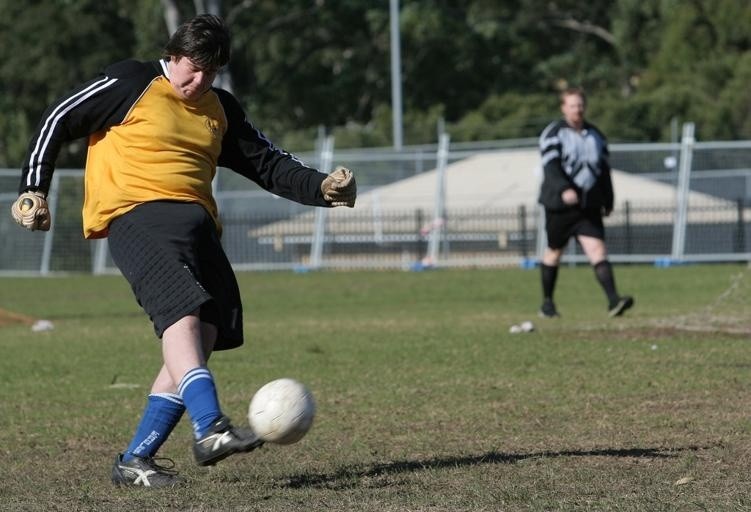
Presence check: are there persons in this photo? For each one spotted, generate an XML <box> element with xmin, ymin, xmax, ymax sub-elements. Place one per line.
<box><xmin>11</xmin><ymin>14</ymin><xmax>357</xmax><ymax>489</ymax></box>
<box><xmin>537</xmin><ymin>89</ymin><xmax>634</xmax><ymax>318</ymax></box>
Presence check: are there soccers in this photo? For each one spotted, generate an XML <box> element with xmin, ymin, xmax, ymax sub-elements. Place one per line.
<box><xmin>248</xmin><ymin>378</ymin><xmax>316</xmax><ymax>444</ymax></box>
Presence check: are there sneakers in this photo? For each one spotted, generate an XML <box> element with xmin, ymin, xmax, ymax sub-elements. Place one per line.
<box><xmin>110</xmin><ymin>452</ymin><xmax>187</xmax><ymax>487</ymax></box>
<box><xmin>541</xmin><ymin>303</ymin><xmax>561</xmax><ymax>320</ymax></box>
<box><xmin>607</xmin><ymin>297</ymin><xmax>633</xmax><ymax>319</ymax></box>
<box><xmin>192</xmin><ymin>415</ymin><xmax>263</xmax><ymax>467</ymax></box>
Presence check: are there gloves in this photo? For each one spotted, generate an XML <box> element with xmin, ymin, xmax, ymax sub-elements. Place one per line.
<box><xmin>10</xmin><ymin>189</ymin><xmax>51</xmax><ymax>232</ymax></box>
<box><xmin>320</xmin><ymin>168</ymin><xmax>356</xmax><ymax>209</ymax></box>
